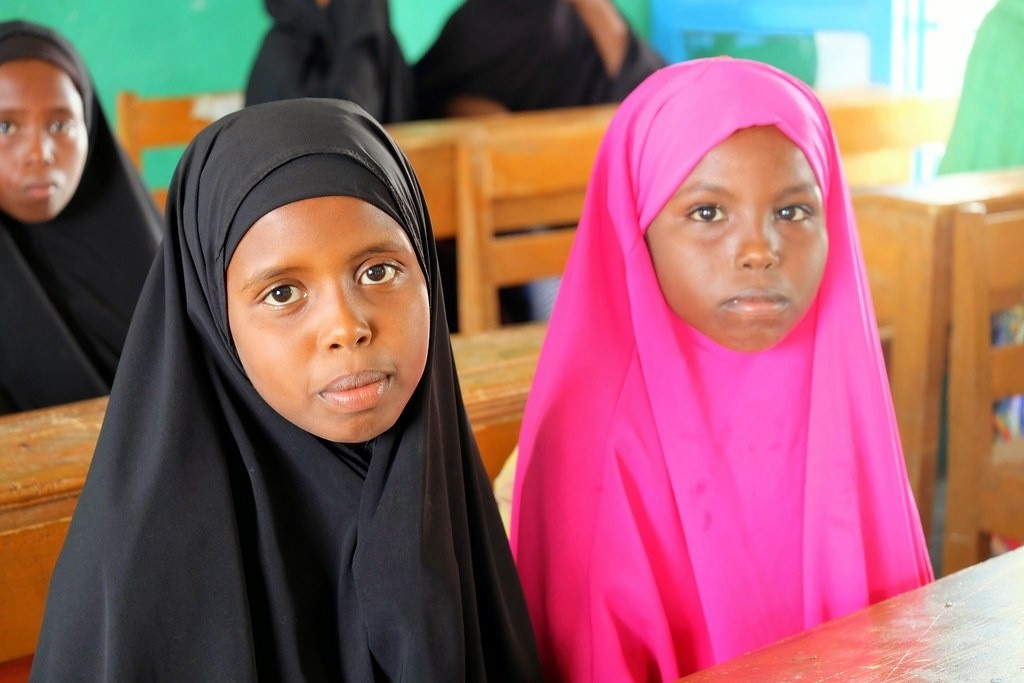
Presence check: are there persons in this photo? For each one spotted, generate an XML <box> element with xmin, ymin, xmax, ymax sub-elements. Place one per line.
<box><xmin>933</xmin><ymin>0</ymin><xmax>1024</xmax><ymax>553</ymax></box>
<box><xmin>0</xmin><ymin>21</ymin><xmax>165</xmax><ymax>415</ymax></box>
<box><xmin>246</xmin><ymin>0</ymin><xmax>411</xmax><ymax>126</ymax></box>
<box><xmin>493</xmin><ymin>57</ymin><xmax>935</xmax><ymax>683</ymax></box>
<box><xmin>30</xmin><ymin>99</ymin><xmax>544</xmax><ymax>683</ymax></box>
<box><xmin>411</xmin><ymin>0</ymin><xmax>668</xmax><ymax>333</ymax></box>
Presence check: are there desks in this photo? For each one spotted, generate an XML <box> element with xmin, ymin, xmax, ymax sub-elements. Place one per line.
<box><xmin>383</xmin><ymin>80</ymin><xmax>954</xmax><ymax>239</ymax></box>
<box><xmin>844</xmin><ymin>164</ymin><xmax>1024</xmax><ymax>542</ymax></box>
<box><xmin>653</xmin><ymin>545</ymin><xmax>1024</xmax><ymax>682</ymax></box>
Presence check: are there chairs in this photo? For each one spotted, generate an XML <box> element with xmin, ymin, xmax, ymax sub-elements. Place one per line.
<box><xmin>938</xmin><ymin>203</ymin><xmax>1023</xmax><ymax>578</ymax></box>
<box><xmin>459</xmin><ymin>119</ymin><xmax>602</xmax><ymax>334</ymax></box>
<box><xmin>113</xmin><ymin>88</ymin><xmax>249</xmax><ymax>217</ymax></box>
<box><xmin>0</xmin><ymin>322</ymin><xmax>570</xmax><ymax>683</ymax></box>
